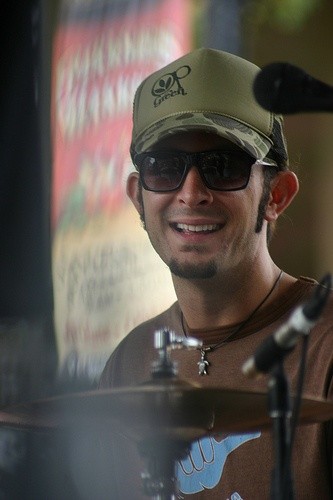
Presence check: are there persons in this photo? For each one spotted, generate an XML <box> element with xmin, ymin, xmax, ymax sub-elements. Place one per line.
<box><xmin>97</xmin><ymin>46</ymin><xmax>333</xmax><ymax>500</ymax></box>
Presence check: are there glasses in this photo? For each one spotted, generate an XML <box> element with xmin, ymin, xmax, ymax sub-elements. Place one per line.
<box><xmin>133</xmin><ymin>148</ymin><xmax>277</xmax><ymax>191</ymax></box>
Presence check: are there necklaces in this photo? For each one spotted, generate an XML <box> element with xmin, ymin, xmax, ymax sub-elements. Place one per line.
<box><xmin>179</xmin><ymin>267</ymin><xmax>285</xmax><ymax>379</ymax></box>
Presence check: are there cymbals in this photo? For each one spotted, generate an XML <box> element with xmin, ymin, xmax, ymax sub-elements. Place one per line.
<box><xmin>1</xmin><ymin>367</ymin><xmax>332</xmax><ymax>438</ymax></box>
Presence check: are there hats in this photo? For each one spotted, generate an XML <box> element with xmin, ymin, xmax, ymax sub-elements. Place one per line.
<box><xmin>130</xmin><ymin>47</ymin><xmax>289</xmax><ymax>172</ymax></box>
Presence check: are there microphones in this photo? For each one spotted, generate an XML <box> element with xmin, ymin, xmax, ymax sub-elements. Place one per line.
<box><xmin>253</xmin><ymin>62</ymin><xmax>333</xmax><ymax>115</ymax></box>
<box><xmin>243</xmin><ymin>296</ymin><xmax>324</xmax><ymax>380</ymax></box>
